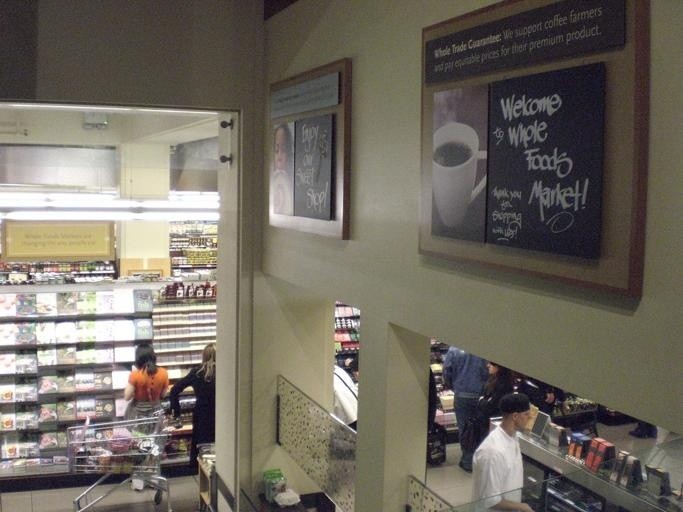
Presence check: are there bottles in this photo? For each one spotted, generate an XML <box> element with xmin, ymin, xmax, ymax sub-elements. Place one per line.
<box><xmin>175</xmin><ymin>284</ymin><xmax>212</xmax><ymax>297</ymax></box>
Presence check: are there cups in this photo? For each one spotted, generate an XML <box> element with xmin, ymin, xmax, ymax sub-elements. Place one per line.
<box><xmin>431</xmin><ymin>122</ymin><xmax>488</xmax><ymax>227</ymax></box>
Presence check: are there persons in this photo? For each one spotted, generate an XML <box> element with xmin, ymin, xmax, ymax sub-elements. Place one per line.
<box><xmin>332</xmin><ymin>345</ymin><xmax>555</xmax><ymax>512</ymax></box>
<box><xmin>124</xmin><ymin>342</ymin><xmax>216</xmax><ymax>491</ymax></box>
<box><xmin>629</xmin><ymin>421</ymin><xmax>657</xmax><ymax>439</ymax></box>
<box><xmin>268</xmin><ymin>123</ymin><xmax>294</xmax><ymax>215</ymax></box>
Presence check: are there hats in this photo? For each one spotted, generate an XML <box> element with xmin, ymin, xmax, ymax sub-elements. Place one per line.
<box><xmin>499</xmin><ymin>393</ymin><xmax>530</xmax><ymax>413</ymax></box>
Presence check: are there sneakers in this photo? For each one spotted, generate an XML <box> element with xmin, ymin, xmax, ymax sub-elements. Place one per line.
<box><xmin>629</xmin><ymin>426</ymin><xmax>657</xmax><ymax>439</ymax></box>
<box><xmin>459</xmin><ymin>461</ymin><xmax>472</xmax><ymax>471</ymax></box>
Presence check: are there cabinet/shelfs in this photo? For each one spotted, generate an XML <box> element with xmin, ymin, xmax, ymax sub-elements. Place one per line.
<box><xmin>171</xmin><ymin>228</ymin><xmax>216</xmax><ymax>272</ymax></box>
<box><xmin>429</xmin><ymin>337</ymin><xmax>539</xmax><ymax>432</ymax></box>
<box><xmin>0</xmin><ymin>276</ymin><xmax>217</xmax><ymax>479</ymax></box>
<box><xmin>487</xmin><ymin>404</ymin><xmax>680</xmax><ymax>512</ymax></box>
<box><xmin>334</xmin><ymin>300</ymin><xmax>360</xmax><ymax>427</ymax></box>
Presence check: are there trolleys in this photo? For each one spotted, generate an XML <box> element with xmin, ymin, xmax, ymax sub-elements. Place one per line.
<box><xmin>65</xmin><ymin>403</ymin><xmax>177</xmax><ymax>512</ymax></box>
